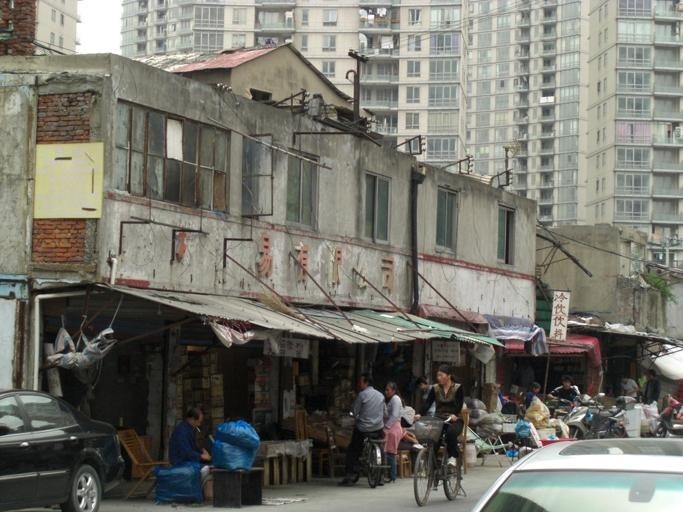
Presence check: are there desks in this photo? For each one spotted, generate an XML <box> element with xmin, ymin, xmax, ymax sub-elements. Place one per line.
<box><xmin>210</xmin><ymin>437</ymin><xmax>312</xmax><ymax>508</ymax></box>
<box><xmin>476</xmin><ymin>422</ymin><xmax>514</xmax><ymax>467</ymax></box>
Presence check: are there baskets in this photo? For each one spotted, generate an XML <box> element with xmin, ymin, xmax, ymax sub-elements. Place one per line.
<box><xmin>414</xmin><ymin>416</ymin><xmax>444</xmax><ymax>443</ymax></box>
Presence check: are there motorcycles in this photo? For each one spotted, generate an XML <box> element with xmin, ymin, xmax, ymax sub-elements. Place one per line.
<box><xmin>551</xmin><ymin>386</ymin><xmax>683</xmax><ymax>439</ymax></box>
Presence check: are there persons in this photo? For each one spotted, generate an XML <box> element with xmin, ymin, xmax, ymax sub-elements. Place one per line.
<box><xmin>414</xmin><ymin>374</ymin><xmax>435</xmax><ymax>416</ymax></box>
<box><xmin>338</xmin><ymin>373</ymin><xmax>388</xmax><ymax>484</ymax></box>
<box><xmin>641</xmin><ymin>368</ymin><xmax>662</xmax><ymax>404</ymax></box>
<box><xmin>526</xmin><ymin>380</ymin><xmax>541</xmax><ymax>410</ymax></box>
<box><xmin>546</xmin><ymin>378</ymin><xmax>579</xmax><ymax>405</ymax></box>
<box><xmin>402</xmin><ymin>426</ymin><xmax>419</xmax><ymax>445</ymax></box>
<box><xmin>616</xmin><ymin>372</ymin><xmax>641</xmax><ymax>399</ymax></box>
<box><xmin>169</xmin><ymin>406</ymin><xmax>214</xmax><ymax>502</ymax></box>
<box><xmin>381</xmin><ymin>380</ymin><xmax>402</xmax><ymax>483</ymax></box>
<box><xmin>413</xmin><ymin>363</ymin><xmax>464</xmax><ymax>491</ymax></box>
<box><xmin>332</xmin><ymin>376</ymin><xmax>357</xmax><ymax>412</ymax></box>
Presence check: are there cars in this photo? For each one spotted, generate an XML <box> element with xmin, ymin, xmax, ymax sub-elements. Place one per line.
<box><xmin>470</xmin><ymin>437</ymin><xmax>683</xmax><ymax>511</ymax></box>
<box><xmin>0</xmin><ymin>388</ymin><xmax>126</xmax><ymax>512</ymax></box>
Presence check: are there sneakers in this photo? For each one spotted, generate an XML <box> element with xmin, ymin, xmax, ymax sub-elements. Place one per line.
<box><xmin>446</xmin><ymin>457</ymin><xmax>457</xmax><ymax>467</ymax></box>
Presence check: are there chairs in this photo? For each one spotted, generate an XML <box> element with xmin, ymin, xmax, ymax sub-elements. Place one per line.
<box><xmin>292</xmin><ymin>408</ymin><xmax>348</xmax><ymax>480</ymax></box>
<box><xmin>466</xmin><ymin>422</ymin><xmax>519</xmax><ymax>467</ymax></box>
<box><xmin>438</xmin><ymin>409</ymin><xmax>470</xmax><ymax>473</ymax></box>
<box><xmin>396</xmin><ymin>449</ymin><xmax>413</xmax><ymax>478</ymax></box>
<box><xmin>115</xmin><ymin>430</ymin><xmax>172</xmax><ymax>501</ymax></box>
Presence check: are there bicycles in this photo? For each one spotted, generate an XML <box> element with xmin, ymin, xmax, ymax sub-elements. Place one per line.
<box><xmin>408</xmin><ymin>412</ymin><xmax>469</xmax><ymax>506</ymax></box>
<box><xmin>349</xmin><ymin>411</ymin><xmax>393</xmax><ymax>489</ymax></box>
